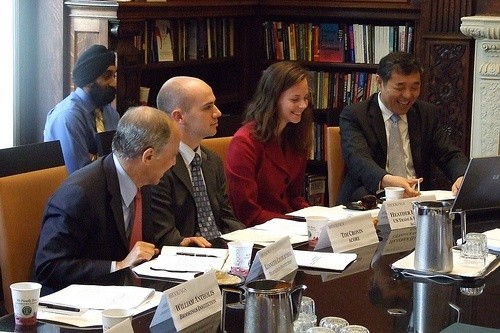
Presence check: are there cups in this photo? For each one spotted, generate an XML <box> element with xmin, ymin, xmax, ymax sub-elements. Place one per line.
<box><xmin>305</xmin><ymin>215</ymin><xmax>329</xmax><ymax>246</ymax></box>
<box><xmin>384</xmin><ymin>187</ymin><xmax>405</xmax><ymax>202</ymax></box>
<box><xmin>9</xmin><ymin>282</ymin><xmax>43</xmax><ymax>326</ymax></box>
<box><xmin>227</xmin><ymin>241</ymin><xmax>254</xmax><ymax>276</ymax></box>
<box><xmin>101</xmin><ymin>308</ymin><xmax>134</xmax><ymax>333</ymax></box>
<box><xmin>293</xmin><ymin>296</ymin><xmax>370</xmax><ymax>333</ymax></box>
<box><xmin>462</xmin><ymin>232</ymin><xmax>489</xmax><ymax>274</ymax></box>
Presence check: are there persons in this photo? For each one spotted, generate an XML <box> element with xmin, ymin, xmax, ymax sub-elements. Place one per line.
<box><xmin>151</xmin><ymin>76</ymin><xmax>247</xmax><ymax>248</ymax></box>
<box><xmin>339</xmin><ymin>51</ymin><xmax>468</xmax><ymax>205</ymax></box>
<box><xmin>43</xmin><ymin>45</ymin><xmax>120</xmax><ymax>173</ymax></box>
<box><xmin>29</xmin><ymin>107</ymin><xmax>212</xmax><ymax>296</ymax></box>
<box><xmin>226</xmin><ymin>62</ymin><xmax>313</xmax><ymax>226</ymax></box>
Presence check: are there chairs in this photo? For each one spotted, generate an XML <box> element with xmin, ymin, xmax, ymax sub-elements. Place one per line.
<box><xmin>200</xmin><ymin>114</ymin><xmax>242</xmax><ymax>195</ymax></box>
<box><xmin>0</xmin><ymin>139</ymin><xmax>70</xmax><ymax>316</ymax></box>
<box><xmin>326</xmin><ymin>108</ymin><xmax>346</xmax><ymax>208</ymax></box>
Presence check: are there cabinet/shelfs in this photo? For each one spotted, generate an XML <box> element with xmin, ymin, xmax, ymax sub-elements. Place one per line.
<box><xmin>64</xmin><ymin>0</ymin><xmax>479</xmax><ymax>205</ymax></box>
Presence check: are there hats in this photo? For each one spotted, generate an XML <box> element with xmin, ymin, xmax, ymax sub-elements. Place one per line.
<box><xmin>72</xmin><ymin>44</ymin><xmax>116</xmax><ymax>89</ymax></box>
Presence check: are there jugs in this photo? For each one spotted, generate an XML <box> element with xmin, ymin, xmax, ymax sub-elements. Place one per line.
<box><xmin>411</xmin><ymin>200</ymin><xmax>467</xmax><ymax>274</ymax></box>
<box><xmin>407</xmin><ymin>275</ymin><xmax>462</xmax><ymax>333</ymax></box>
<box><xmin>220</xmin><ymin>279</ymin><xmax>307</xmax><ymax>333</ymax></box>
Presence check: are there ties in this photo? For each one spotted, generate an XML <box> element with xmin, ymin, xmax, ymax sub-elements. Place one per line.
<box><xmin>190</xmin><ymin>153</ymin><xmax>222</xmax><ymax>242</ymax></box>
<box><xmin>388</xmin><ymin>113</ymin><xmax>408</xmax><ymax>179</ymax></box>
<box><xmin>128</xmin><ymin>188</ymin><xmax>143</xmax><ymax>252</ymax></box>
<box><xmin>129</xmin><ymin>261</ymin><xmax>143</xmax><ymax>287</ymax></box>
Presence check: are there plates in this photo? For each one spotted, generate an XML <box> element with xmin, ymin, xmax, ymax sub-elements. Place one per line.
<box><xmin>194</xmin><ymin>270</ymin><xmax>245</xmax><ymax>288</ymax></box>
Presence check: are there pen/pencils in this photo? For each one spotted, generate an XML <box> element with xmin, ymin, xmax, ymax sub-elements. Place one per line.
<box><xmin>177</xmin><ymin>252</ymin><xmax>216</xmax><ymax>257</ymax></box>
<box><xmin>39</xmin><ymin>303</ymin><xmax>80</xmax><ymax>312</ymax></box>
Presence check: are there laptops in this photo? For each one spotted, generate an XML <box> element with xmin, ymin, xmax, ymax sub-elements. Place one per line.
<box><xmin>412</xmin><ymin>155</ymin><xmax>500</xmax><ymax>219</ymax></box>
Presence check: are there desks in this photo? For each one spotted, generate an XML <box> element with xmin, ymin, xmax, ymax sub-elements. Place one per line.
<box><xmin>0</xmin><ymin>210</ymin><xmax>500</xmax><ymax>333</ymax></box>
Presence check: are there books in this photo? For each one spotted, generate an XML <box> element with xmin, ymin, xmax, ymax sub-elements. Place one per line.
<box><xmin>263</xmin><ymin>21</ymin><xmax>414</xmax><ymax>64</ymax></box>
<box><xmin>308</xmin><ymin>71</ymin><xmax>381</xmax><ymax>109</ymax></box>
<box><xmin>305</xmin><ymin>174</ymin><xmax>325</xmax><ymax>205</ymax></box>
<box><xmin>308</xmin><ymin>121</ymin><xmax>338</xmax><ymax>161</ymax></box>
<box><xmin>151</xmin><ymin>19</ymin><xmax>234</xmax><ymax>62</ymax></box>
